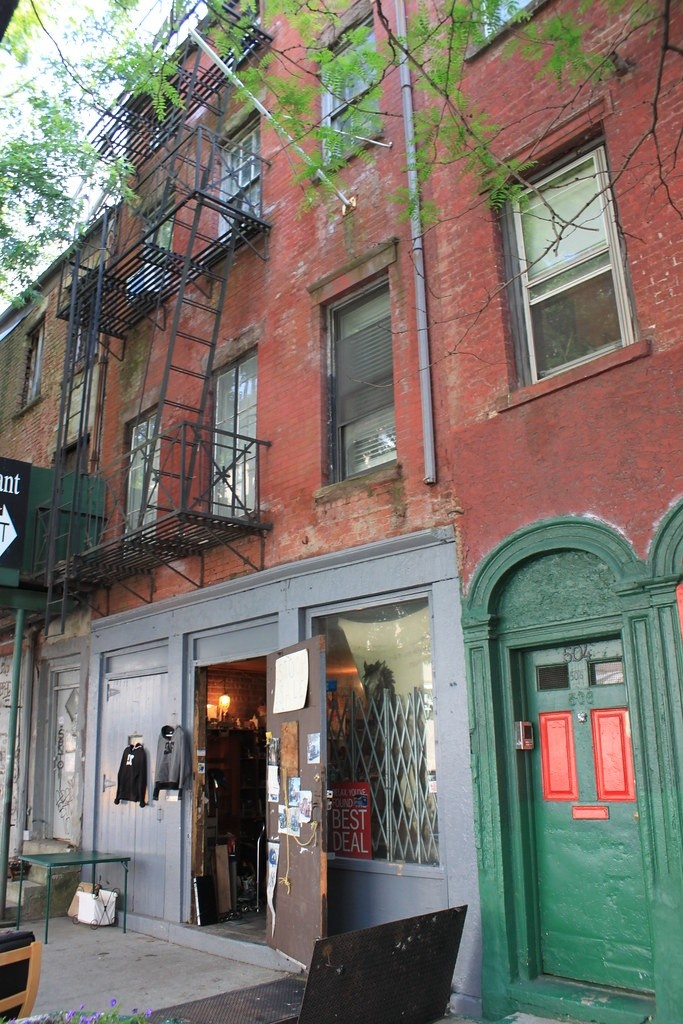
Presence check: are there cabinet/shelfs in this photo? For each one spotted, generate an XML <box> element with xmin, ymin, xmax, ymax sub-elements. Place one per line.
<box><xmin>205</xmin><ymin>728</ymin><xmax>266</xmax><ymax>857</ymax></box>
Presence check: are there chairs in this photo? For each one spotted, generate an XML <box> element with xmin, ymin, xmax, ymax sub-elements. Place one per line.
<box><xmin>0</xmin><ymin>930</ymin><xmax>41</xmax><ymax>1022</ymax></box>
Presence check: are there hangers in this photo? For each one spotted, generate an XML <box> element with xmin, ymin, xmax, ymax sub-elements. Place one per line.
<box><xmin>129</xmin><ymin>736</ymin><xmax>141</xmax><ymax>751</ymax></box>
<box><xmin>163</xmin><ymin>713</ymin><xmax>178</xmax><ymax>737</ymax></box>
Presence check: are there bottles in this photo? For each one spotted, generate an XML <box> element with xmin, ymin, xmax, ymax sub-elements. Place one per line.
<box><xmin>242</xmin><ymin>877</ymin><xmax>249</xmax><ymax>890</ymax></box>
<box><xmin>250</xmin><ymin>714</ymin><xmax>258</xmax><ymax>730</ymax></box>
<box><xmin>236</xmin><ymin>718</ymin><xmax>241</xmax><ymax>727</ymax></box>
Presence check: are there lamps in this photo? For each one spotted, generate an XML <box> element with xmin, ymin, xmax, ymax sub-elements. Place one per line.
<box><xmin>218</xmin><ymin>694</ymin><xmax>230</xmax><ymax>722</ymax></box>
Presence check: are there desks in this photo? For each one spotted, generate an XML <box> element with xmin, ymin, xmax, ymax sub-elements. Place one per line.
<box><xmin>16</xmin><ymin>850</ymin><xmax>130</xmax><ymax>944</ymax></box>
